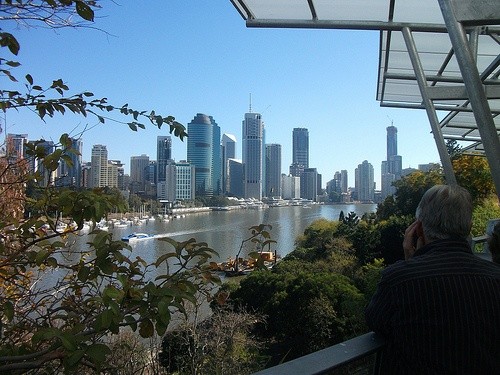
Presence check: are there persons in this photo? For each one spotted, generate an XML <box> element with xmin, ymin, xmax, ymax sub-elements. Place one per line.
<box><xmin>369</xmin><ymin>184</ymin><xmax>500</xmax><ymax>375</ymax></box>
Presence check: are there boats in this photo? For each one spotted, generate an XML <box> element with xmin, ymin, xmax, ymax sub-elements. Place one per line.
<box><xmin>226</xmin><ymin>255</ymin><xmax>281</xmax><ymax>275</ymax></box>
<box><xmin>121</xmin><ymin>232</ymin><xmax>149</xmax><ymax>242</ymax></box>
<box><xmin>42</xmin><ymin>214</ymin><xmax>185</xmax><ymax>231</ymax></box>
